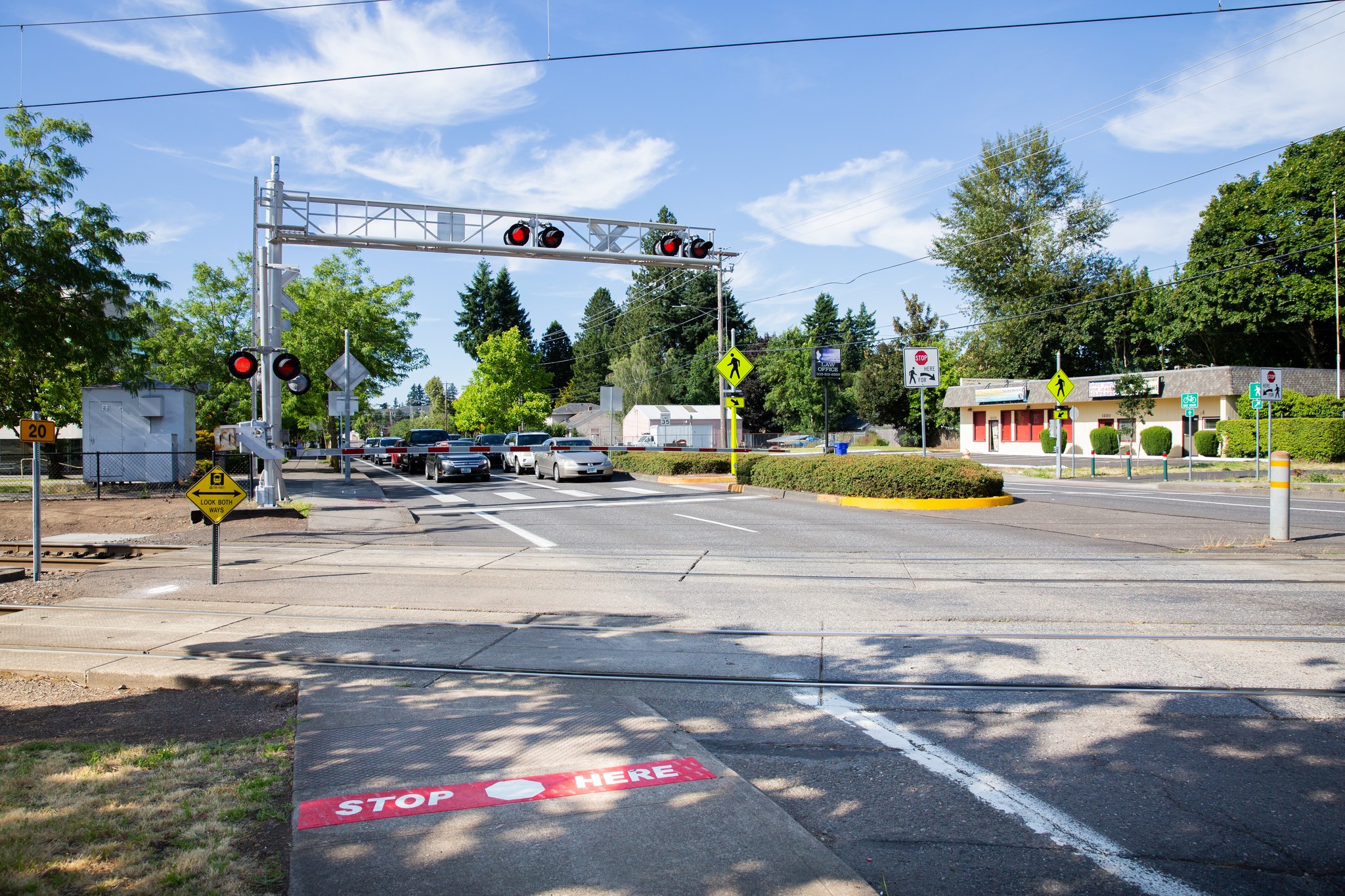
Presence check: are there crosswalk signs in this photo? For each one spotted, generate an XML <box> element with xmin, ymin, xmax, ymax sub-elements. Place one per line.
<box><xmin>1046</xmin><ymin>369</ymin><xmax>1075</xmax><ymax>404</ymax></box>
<box><xmin>1249</xmin><ymin>383</ymin><xmax>1261</xmax><ymax>399</ymax></box>
<box><xmin>713</xmin><ymin>346</ymin><xmax>754</xmax><ymax>388</ymax></box>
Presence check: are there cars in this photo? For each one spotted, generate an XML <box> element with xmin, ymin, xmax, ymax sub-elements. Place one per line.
<box><xmin>425</xmin><ymin>440</ymin><xmax>491</xmax><ymax>483</ymax></box>
<box><xmin>374</xmin><ymin>437</ymin><xmax>403</xmax><ymax>466</ymax></box>
<box><xmin>337</xmin><ymin>438</ymin><xmax>350</xmax><ymax>449</ymax></box>
<box><xmin>370</xmin><ymin>437</ymin><xmax>384</xmax><ymax>462</ymax></box>
<box><xmin>447</xmin><ymin>433</ymin><xmax>476</xmax><ymax>444</ymax></box>
<box><xmin>534</xmin><ymin>437</ymin><xmax>613</xmax><ymax>483</ymax></box>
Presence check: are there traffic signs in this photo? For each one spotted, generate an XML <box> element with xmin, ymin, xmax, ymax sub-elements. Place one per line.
<box><xmin>185</xmin><ymin>465</ymin><xmax>247</xmax><ymax>524</ymax></box>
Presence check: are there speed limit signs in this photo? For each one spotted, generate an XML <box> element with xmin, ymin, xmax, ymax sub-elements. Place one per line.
<box><xmin>660</xmin><ymin>413</ymin><xmax>671</xmax><ymax>426</ymax></box>
<box><xmin>19</xmin><ymin>418</ymin><xmax>55</xmax><ymax>443</ymax></box>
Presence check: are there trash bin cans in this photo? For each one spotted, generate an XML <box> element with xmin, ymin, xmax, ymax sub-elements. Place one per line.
<box><xmin>833</xmin><ymin>442</ymin><xmax>850</xmax><ymax>455</ymax></box>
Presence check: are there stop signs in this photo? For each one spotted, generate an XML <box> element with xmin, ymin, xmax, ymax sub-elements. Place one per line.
<box><xmin>1260</xmin><ymin>368</ymin><xmax>1282</xmax><ymax>401</ymax></box>
<box><xmin>903</xmin><ymin>347</ymin><xmax>940</xmax><ymax>388</ymax></box>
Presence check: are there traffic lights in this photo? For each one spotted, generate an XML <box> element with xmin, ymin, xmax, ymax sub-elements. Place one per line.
<box><xmin>228</xmin><ymin>351</ymin><xmax>258</xmax><ymax>379</ymax></box>
<box><xmin>683</xmin><ymin>238</ymin><xmax>713</xmax><ymax>259</ymax></box>
<box><xmin>503</xmin><ymin>223</ymin><xmax>530</xmax><ymax>246</ymax></box>
<box><xmin>536</xmin><ymin>227</ymin><xmax>564</xmax><ymax>249</ymax></box>
<box><xmin>653</xmin><ymin>235</ymin><xmax>682</xmax><ymax>257</ymax></box>
<box><xmin>273</xmin><ymin>353</ymin><xmax>302</xmax><ymax>381</ymax></box>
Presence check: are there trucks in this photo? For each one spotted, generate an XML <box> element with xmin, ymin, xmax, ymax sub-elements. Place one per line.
<box><xmin>359</xmin><ymin>438</ymin><xmax>378</xmax><ymax>460</ymax></box>
<box><xmin>630</xmin><ymin>425</ymin><xmax>713</xmax><ymax>450</ymax></box>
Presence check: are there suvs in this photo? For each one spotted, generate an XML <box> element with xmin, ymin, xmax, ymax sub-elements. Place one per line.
<box><xmin>401</xmin><ymin>429</ymin><xmax>453</xmax><ymax>475</ymax></box>
<box><xmin>474</xmin><ymin>433</ymin><xmax>507</xmax><ymax>468</ymax></box>
<box><xmin>500</xmin><ymin>431</ymin><xmax>552</xmax><ymax>475</ymax></box>
